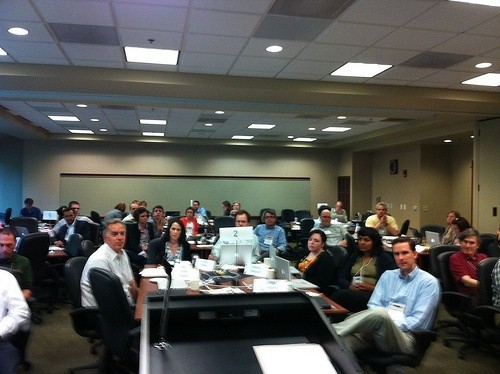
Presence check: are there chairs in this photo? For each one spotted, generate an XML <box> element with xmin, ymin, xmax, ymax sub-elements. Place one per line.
<box><xmin>0</xmin><ymin>208</ymin><xmax>500</xmax><ymax>374</ymax></box>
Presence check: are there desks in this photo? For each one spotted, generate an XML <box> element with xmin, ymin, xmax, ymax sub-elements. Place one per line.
<box><xmin>186</xmin><ymin>241</ymin><xmax>214</xmax><ymax>259</ymax></box>
<box><xmin>134</xmin><ymin>264</ymin><xmax>349</xmax><ymax>321</ymax></box>
<box><xmin>47</xmin><ymin>245</ymin><xmax>68</xmax><ymax>259</ymax></box>
<box><xmin>352</xmin><ymin>234</ymin><xmax>430</xmax><ymax>256</ymax></box>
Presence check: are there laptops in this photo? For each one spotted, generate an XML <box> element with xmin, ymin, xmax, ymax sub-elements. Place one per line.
<box><xmin>425</xmin><ymin>231</ymin><xmax>439</xmax><ymax>246</ymax></box>
<box><xmin>219</xmin><ymin>243</ymin><xmax>253</xmax><ymax>266</ymax></box>
<box><xmin>15</xmin><ymin>227</ymin><xmax>28</xmax><ymax>236</ymax></box>
<box><xmin>275</xmin><ymin>256</ymin><xmax>320</xmax><ymax>289</ymax></box>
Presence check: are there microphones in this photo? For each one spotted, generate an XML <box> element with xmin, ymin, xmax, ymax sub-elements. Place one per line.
<box><xmin>157</xmin><ymin>258</ymin><xmax>171</xmax><ymax>344</ymax></box>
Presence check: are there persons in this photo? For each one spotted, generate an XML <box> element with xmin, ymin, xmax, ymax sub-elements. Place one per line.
<box><xmin>0</xmin><ymin>198</ymin><xmax>500</xmax><ymax>374</ymax></box>
<box><xmin>331</xmin><ymin>237</ymin><xmax>440</xmax><ymax>359</ymax></box>
<box><xmin>81</xmin><ymin>219</ymin><xmax>139</xmax><ymax>336</ymax></box>
